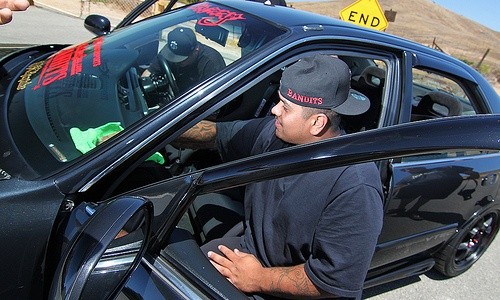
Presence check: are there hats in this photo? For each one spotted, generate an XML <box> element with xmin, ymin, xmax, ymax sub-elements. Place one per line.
<box><xmin>279</xmin><ymin>55</ymin><xmax>371</xmax><ymax>116</ymax></box>
<box><xmin>159</xmin><ymin>26</ymin><xmax>197</xmax><ymax>63</ymax></box>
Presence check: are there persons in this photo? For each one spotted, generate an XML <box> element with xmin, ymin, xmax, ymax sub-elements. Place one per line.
<box><xmin>386</xmin><ymin>164</ymin><xmax>479</xmax><ymax>221</ymax></box>
<box><xmin>0</xmin><ymin>0</ymin><xmax>31</xmax><ymax>24</ymax></box>
<box><xmin>97</xmin><ymin>54</ymin><xmax>384</xmax><ymax>300</ymax></box>
<box><xmin>141</xmin><ymin>27</ymin><xmax>226</xmax><ymax>96</ymax></box>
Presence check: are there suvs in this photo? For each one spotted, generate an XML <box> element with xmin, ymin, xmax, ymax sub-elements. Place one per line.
<box><xmin>0</xmin><ymin>0</ymin><xmax>499</xmax><ymax>299</ymax></box>
